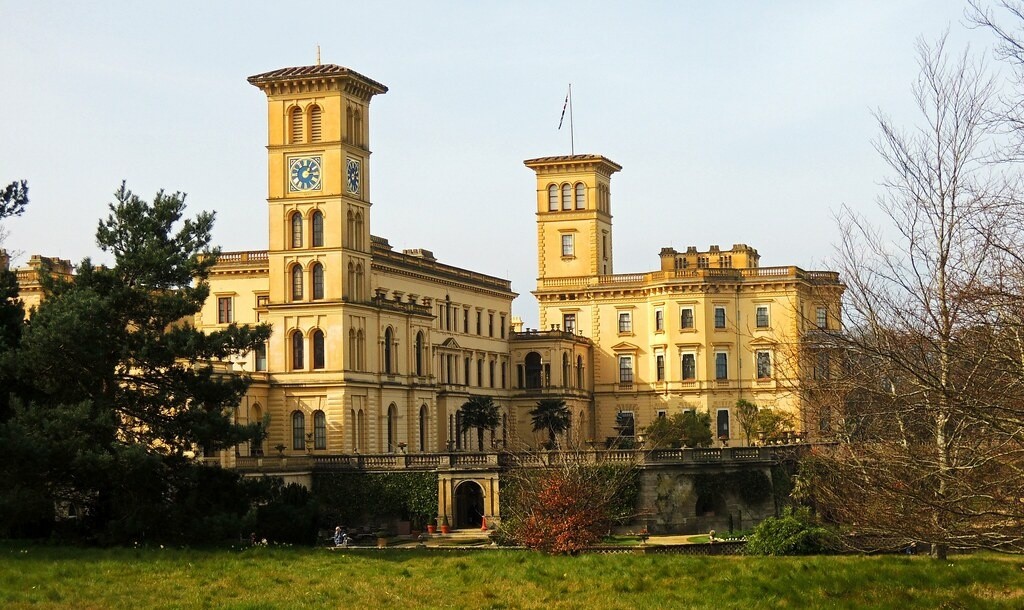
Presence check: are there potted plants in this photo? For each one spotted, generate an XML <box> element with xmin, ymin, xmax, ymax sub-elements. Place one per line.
<box><xmin>426</xmin><ymin>513</ymin><xmax>438</xmax><ymax>533</ymax></box>
<box><xmin>440</xmin><ymin>514</ymin><xmax>449</xmax><ymax>533</ymax></box>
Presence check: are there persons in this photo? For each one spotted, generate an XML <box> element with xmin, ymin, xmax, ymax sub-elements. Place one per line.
<box><xmin>334</xmin><ymin>527</ymin><xmax>344</xmax><ymax>546</ymax></box>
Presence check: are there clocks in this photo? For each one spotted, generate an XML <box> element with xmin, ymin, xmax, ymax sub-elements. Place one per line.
<box><xmin>289</xmin><ymin>156</ymin><xmax>323</xmax><ymax>191</ymax></box>
<box><xmin>345</xmin><ymin>158</ymin><xmax>360</xmax><ymax>195</ymax></box>
